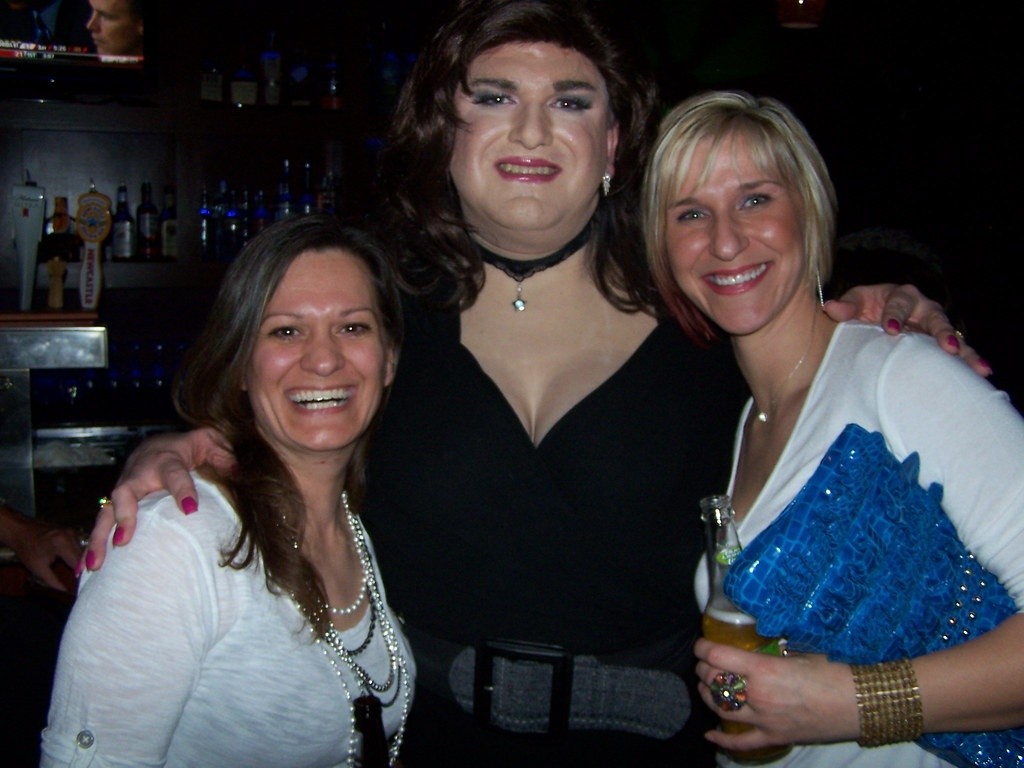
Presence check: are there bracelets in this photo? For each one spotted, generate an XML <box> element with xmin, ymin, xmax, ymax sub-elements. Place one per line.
<box><xmin>850</xmin><ymin>658</ymin><xmax>924</xmax><ymax>748</ymax></box>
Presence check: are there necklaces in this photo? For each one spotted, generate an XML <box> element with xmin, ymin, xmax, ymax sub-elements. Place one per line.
<box><xmin>760</xmin><ymin>334</ymin><xmax>812</xmax><ymax>422</ymax></box>
<box><xmin>282</xmin><ymin>490</ymin><xmax>408</xmax><ymax>767</ymax></box>
<box><xmin>467</xmin><ymin>206</ymin><xmax>601</xmax><ymax>310</ymax></box>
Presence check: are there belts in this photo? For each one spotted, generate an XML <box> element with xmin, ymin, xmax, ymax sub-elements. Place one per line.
<box><xmin>413</xmin><ymin>631</ymin><xmax>690</xmax><ymax>741</ymax></box>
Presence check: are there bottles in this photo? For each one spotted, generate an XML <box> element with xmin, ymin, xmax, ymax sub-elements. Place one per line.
<box><xmin>36</xmin><ymin>197</ymin><xmax>83</xmax><ymax>261</ymax></box>
<box><xmin>299</xmin><ymin>160</ymin><xmax>314</xmax><ymax>215</ymax></box>
<box><xmin>696</xmin><ymin>495</ymin><xmax>792</xmax><ymax>766</ymax></box>
<box><xmin>198</xmin><ymin>181</ymin><xmax>212</xmax><ymax>255</ymax></box>
<box><xmin>110</xmin><ymin>184</ymin><xmax>135</xmax><ymax>263</ymax></box>
<box><xmin>270</xmin><ymin>159</ymin><xmax>296</xmax><ymax>219</ymax></box>
<box><xmin>316</xmin><ymin>138</ymin><xmax>349</xmax><ymax>214</ymax></box>
<box><xmin>160</xmin><ymin>173</ymin><xmax>179</xmax><ymax>258</ymax></box>
<box><xmin>136</xmin><ymin>178</ymin><xmax>161</xmax><ymax>262</ymax></box>
<box><xmin>378</xmin><ymin>20</ymin><xmax>418</xmax><ymax>104</ymax></box>
<box><xmin>320</xmin><ymin>50</ymin><xmax>343</xmax><ymax>109</ymax></box>
<box><xmin>197</xmin><ymin>33</ymin><xmax>289</xmax><ymax>111</ymax></box>
<box><xmin>216</xmin><ymin>177</ymin><xmax>270</xmax><ymax>244</ymax></box>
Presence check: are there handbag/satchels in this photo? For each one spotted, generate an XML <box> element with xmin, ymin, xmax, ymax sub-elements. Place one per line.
<box><xmin>723</xmin><ymin>422</ymin><xmax>1024</xmax><ymax>768</ymax></box>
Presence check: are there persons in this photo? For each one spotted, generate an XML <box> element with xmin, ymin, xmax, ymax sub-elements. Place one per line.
<box><xmin>0</xmin><ymin>0</ymin><xmax>97</xmax><ymax>53</ymax></box>
<box><xmin>76</xmin><ymin>0</ymin><xmax>993</xmax><ymax>768</ymax></box>
<box><xmin>640</xmin><ymin>89</ymin><xmax>1024</xmax><ymax>768</ymax></box>
<box><xmin>86</xmin><ymin>0</ymin><xmax>144</xmax><ymax>54</ymax></box>
<box><xmin>0</xmin><ymin>500</ymin><xmax>90</xmax><ymax>592</ymax></box>
<box><xmin>37</xmin><ymin>213</ymin><xmax>419</xmax><ymax>766</ymax></box>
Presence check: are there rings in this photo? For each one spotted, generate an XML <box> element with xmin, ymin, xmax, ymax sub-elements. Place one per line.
<box><xmin>955</xmin><ymin>331</ymin><xmax>962</xmax><ymax>339</ymax></box>
<box><xmin>711</xmin><ymin>673</ymin><xmax>747</xmax><ymax>710</ymax></box>
<box><xmin>80</xmin><ymin>534</ymin><xmax>90</xmax><ymax>545</ymax></box>
<box><xmin>98</xmin><ymin>496</ymin><xmax>113</xmax><ymax>508</ymax></box>
